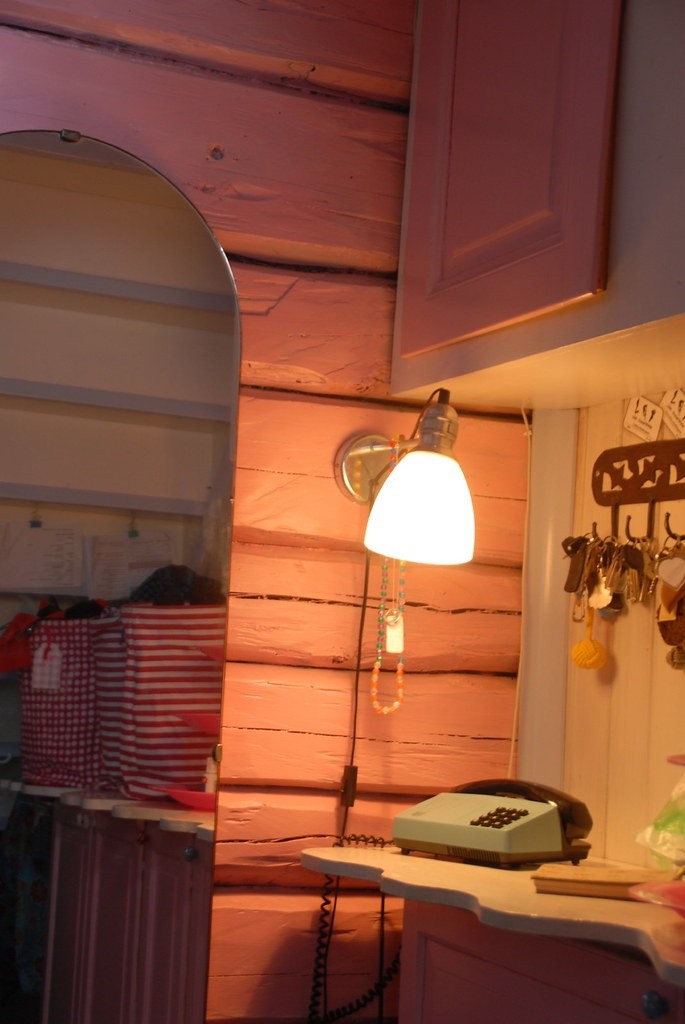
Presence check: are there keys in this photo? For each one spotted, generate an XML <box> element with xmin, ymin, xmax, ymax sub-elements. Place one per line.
<box><xmin>563</xmin><ymin>527</ymin><xmax>685</xmax><ymax>610</ymax></box>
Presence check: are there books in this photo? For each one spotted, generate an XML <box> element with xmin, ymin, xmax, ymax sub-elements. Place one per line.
<box><xmin>531</xmin><ymin>861</ymin><xmax>683</xmax><ymax>902</ymax></box>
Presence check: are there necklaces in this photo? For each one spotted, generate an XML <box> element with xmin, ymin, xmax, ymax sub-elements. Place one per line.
<box><xmin>371</xmin><ymin>436</ymin><xmax>409</xmax><ymax>715</ymax></box>
<box><xmin>346</xmin><ymin>443</ymin><xmax>362</xmax><ymax>499</ymax></box>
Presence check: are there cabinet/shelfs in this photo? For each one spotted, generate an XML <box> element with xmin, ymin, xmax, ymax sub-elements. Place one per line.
<box><xmin>0</xmin><ymin>782</ymin><xmax>215</xmax><ymax>1024</ymax></box>
<box><xmin>301</xmin><ymin>848</ymin><xmax>685</xmax><ymax>1024</ymax></box>
<box><xmin>401</xmin><ymin>0</ymin><xmax>626</xmax><ymax>360</ymax></box>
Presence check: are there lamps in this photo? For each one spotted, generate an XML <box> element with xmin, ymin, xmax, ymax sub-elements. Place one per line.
<box><xmin>333</xmin><ymin>387</ymin><xmax>475</xmax><ymax>565</ymax></box>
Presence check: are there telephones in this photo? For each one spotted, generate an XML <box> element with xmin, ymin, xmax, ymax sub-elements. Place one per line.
<box><xmin>391</xmin><ymin>778</ymin><xmax>594</xmax><ymax>870</ymax></box>
